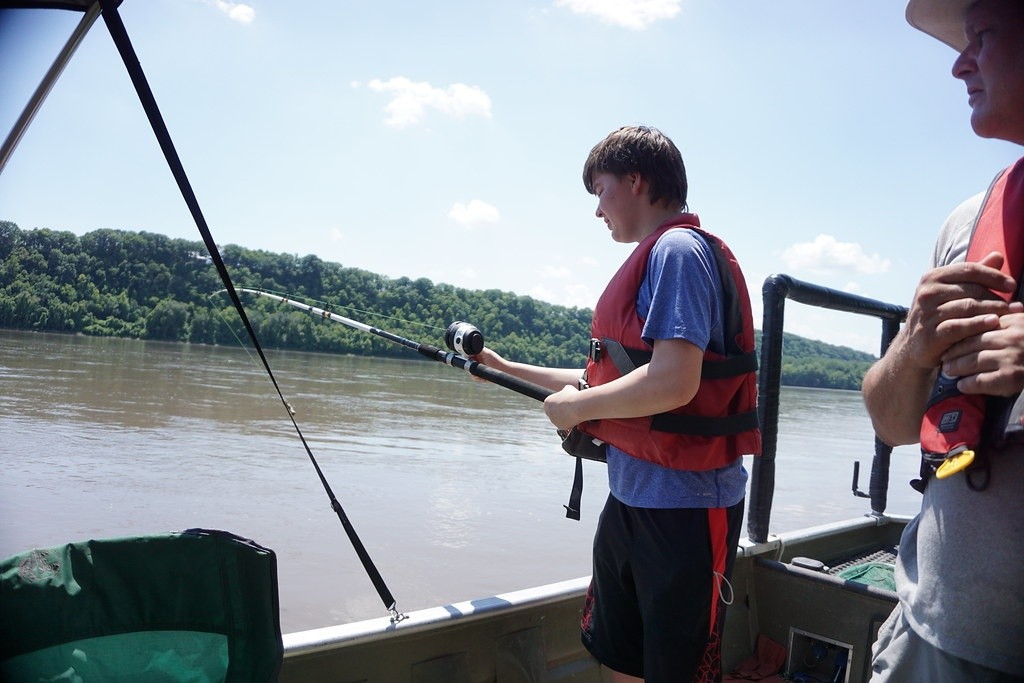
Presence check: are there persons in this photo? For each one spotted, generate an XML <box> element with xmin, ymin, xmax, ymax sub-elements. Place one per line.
<box><xmin>861</xmin><ymin>0</ymin><xmax>1024</xmax><ymax>683</ymax></box>
<box><xmin>470</xmin><ymin>125</ymin><xmax>763</xmax><ymax>683</ymax></box>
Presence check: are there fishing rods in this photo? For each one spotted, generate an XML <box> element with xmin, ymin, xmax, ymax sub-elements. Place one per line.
<box><xmin>208</xmin><ymin>289</ymin><xmax>553</xmax><ymax>400</ymax></box>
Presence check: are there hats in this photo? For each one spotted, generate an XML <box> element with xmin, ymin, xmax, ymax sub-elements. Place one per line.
<box><xmin>905</xmin><ymin>0</ymin><xmax>977</xmax><ymax>53</ymax></box>
